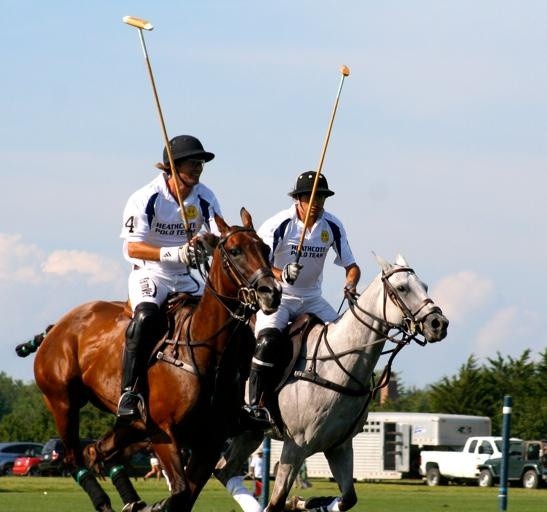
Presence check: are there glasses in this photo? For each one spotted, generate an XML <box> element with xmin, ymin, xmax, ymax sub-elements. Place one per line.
<box><xmin>186</xmin><ymin>159</ymin><xmax>205</xmax><ymax>168</ymax></box>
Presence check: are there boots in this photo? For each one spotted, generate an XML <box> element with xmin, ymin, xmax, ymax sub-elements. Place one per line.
<box><xmin>249</xmin><ymin>366</ymin><xmax>275</xmax><ymax>420</ymax></box>
<box><xmin>117</xmin><ymin>347</ymin><xmax>145</xmax><ymax>416</ymax></box>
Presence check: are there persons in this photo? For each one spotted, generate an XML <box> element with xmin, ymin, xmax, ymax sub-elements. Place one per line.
<box><xmin>236</xmin><ymin>168</ymin><xmax>364</xmax><ymax>421</ymax></box>
<box><xmin>116</xmin><ymin>127</ymin><xmax>223</xmax><ymax>429</ymax></box>
<box><xmin>143</xmin><ymin>453</ymin><xmax>160</xmax><ymax>482</ymax></box>
<box><xmin>250</xmin><ymin>448</ymin><xmax>264</xmax><ymax>500</ymax></box>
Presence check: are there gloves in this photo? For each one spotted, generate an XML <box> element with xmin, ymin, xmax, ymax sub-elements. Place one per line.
<box><xmin>159</xmin><ymin>234</ymin><xmax>208</xmax><ymax>264</ymax></box>
<box><xmin>281</xmin><ymin>262</ymin><xmax>304</xmax><ymax>286</ymax></box>
<box><xmin>344</xmin><ymin>282</ymin><xmax>356</xmax><ymax>298</ymax></box>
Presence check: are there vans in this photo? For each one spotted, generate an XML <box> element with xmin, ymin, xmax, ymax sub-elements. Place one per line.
<box><xmin>0</xmin><ymin>436</ymin><xmax>98</xmax><ymax>478</ymax></box>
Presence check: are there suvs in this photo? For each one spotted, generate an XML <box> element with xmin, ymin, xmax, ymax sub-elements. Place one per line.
<box><xmin>478</xmin><ymin>444</ymin><xmax>547</xmax><ymax>489</ymax></box>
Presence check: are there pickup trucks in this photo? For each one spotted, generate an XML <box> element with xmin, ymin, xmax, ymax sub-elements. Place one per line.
<box><xmin>418</xmin><ymin>435</ymin><xmax>524</xmax><ymax>486</ymax></box>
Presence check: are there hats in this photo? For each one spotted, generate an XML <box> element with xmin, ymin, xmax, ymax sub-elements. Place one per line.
<box><xmin>162</xmin><ymin>134</ymin><xmax>215</xmax><ymax>165</ymax></box>
<box><xmin>288</xmin><ymin>170</ymin><xmax>335</xmax><ymax>197</ymax></box>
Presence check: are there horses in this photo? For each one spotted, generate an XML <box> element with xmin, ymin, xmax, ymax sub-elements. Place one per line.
<box><xmin>15</xmin><ymin>206</ymin><xmax>283</xmax><ymax>512</ymax></box>
<box><xmin>211</xmin><ymin>249</ymin><xmax>449</xmax><ymax>512</ymax></box>
